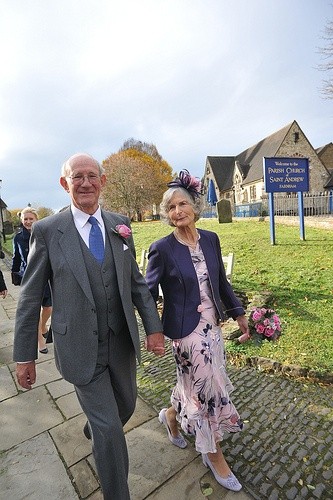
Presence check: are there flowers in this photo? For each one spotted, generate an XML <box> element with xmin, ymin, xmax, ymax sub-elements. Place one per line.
<box><xmin>223</xmin><ymin>295</ymin><xmax>281</xmax><ymax>347</ymax></box>
<box><xmin>110</xmin><ymin>224</ymin><xmax>132</xmax><ymax>247</ymax></box>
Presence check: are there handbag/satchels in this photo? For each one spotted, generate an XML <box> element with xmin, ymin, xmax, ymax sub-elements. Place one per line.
<box><xmin>12</xmin><ymin>272</ymin><xmax>20</xmax><ymax>285</ymax></box>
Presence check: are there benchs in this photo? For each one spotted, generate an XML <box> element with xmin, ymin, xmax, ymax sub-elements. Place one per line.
<box><xmin>140</xmin><ymin>249</ymin><xmax>234</xmax><ymax>297</ymax></box>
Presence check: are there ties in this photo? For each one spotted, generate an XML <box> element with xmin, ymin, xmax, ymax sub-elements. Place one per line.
<box><xmin>88</xmin><ymin>216</ymin><xmax>104</xmax><ymax>267</ymax></box>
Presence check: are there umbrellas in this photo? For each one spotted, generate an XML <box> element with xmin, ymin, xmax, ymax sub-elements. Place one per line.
<box><xmin>207</xmin><ymin>179</ymin><xmax>218</xmax><ymax>218</ymax></box>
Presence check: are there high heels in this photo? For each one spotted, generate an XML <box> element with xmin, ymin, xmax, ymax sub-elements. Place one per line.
<box><xmin>158</xmin><ymin>408</ymin><xmax>187</xmax><ymax>449</ymax></box>
<box><xmin>202</xmin><ymin>448</ymin><xmax>243</xmax><ymax>492</ymax></box>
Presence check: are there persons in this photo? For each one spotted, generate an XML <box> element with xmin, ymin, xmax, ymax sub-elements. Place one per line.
<box><xmin>145</xmin><ymin>168</ymin><xmax>250</xmax><ymax>490</ymax></box>
<box><xmin>10</xmin><ymin>207</ymin><xmax>52</xmax><ymax>354</ymax></box>
<box><xmin>0</xmin><ymin>250</ymin><xmax>7</xmax><ymax>300</ymax></box>
<box><xmin>12</xmin><ymin>154</ymin><xmax>165</xmax><ymax>500</ymax></box>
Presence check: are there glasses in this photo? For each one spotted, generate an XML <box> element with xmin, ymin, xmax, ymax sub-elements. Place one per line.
<box><xmin>66</xmin><ymin>173</ymin><xmax>101</xmax><ymax>185</ymax></box>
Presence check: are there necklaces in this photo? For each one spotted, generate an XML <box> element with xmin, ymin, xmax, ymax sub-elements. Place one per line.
<box><xmin>175</xmin><ymin>226</ymin><xmax>200</xmax><ymax>248</ymax></box>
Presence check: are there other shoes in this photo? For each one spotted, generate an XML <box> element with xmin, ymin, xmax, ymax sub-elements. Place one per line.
<box><xmin>43</xmin><ymin>331</ymin><xmax>50</xmax><ymax>338</ymax></box>
<box><xmin>40</xmin><ymin>347</ymin><xmax>48</xmax><ymax>353</ymax></box>
